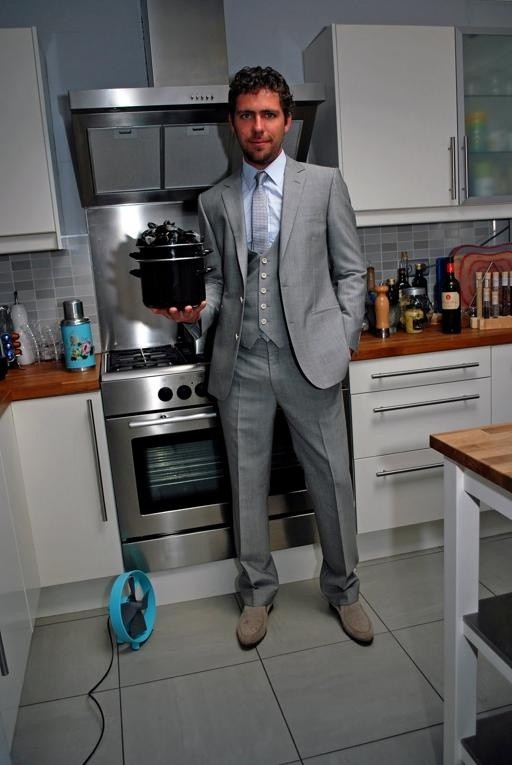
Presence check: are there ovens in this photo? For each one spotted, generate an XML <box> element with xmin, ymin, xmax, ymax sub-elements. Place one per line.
<box><xmin>105</xmin><ymin>389</ymin><xmax>358</xmax><ymax>574</ymax></box>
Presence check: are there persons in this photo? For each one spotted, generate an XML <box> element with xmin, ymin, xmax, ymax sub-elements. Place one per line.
<box><xmin>151</xmin><ymin>66</ymin><xmax>375</xmax><ymax>650</ymax></box>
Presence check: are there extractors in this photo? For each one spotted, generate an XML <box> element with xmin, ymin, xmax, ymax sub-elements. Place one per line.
<box><xmin>66</xmin><ymin>1</ymin><xmax>326</xmax><ymax>209</ymax></box>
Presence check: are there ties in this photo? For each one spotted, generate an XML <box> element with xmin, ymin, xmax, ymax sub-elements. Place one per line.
<box><xmin>251</xmin><ymin>172</ymin><xmax>269</xmax><ymax>255</ymax></box>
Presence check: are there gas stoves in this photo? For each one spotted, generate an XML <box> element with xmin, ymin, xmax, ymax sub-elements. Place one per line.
<box><xmin>100</xmin><ymin>321</ymin><xmax>216</xmax><ymax>419</ymax></box>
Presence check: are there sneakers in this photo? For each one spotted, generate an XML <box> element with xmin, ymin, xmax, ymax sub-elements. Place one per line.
<box><xmin>236</xmin><ymin>602</ymin><xmax>274</xmax><ymax>650</ymax></box>
<box><xmin>327</xmin><ymin>595</ymin><xmax>374</xmax><ymax>647</ymax></box>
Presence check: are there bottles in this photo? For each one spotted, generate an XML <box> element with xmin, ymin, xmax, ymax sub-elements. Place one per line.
<box><xmin>467</xmin><ymin>112</ymin><xmax>486</xmax><ymax>151</ymax></box>
<box><xmin>474</xmin><ymin>271</ymin><xmax>512</xmax><ymax>319</ymax></box>
<box><xmin>366</xmin><ymin>251</ymin><xmax>461</xmax><ymax>338</ymax></box>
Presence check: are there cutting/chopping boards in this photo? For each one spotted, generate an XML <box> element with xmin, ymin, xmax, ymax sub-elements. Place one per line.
<box><xmin>451</xmin><ymin>242</ymin><xmax>512</xmax><ymax>313</ymax></box>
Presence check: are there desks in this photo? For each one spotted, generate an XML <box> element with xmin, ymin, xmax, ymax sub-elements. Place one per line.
<box><xmin>430</xmin><ymin>424</ymin><xmax>511</xmax><ymax>764</ymax></box>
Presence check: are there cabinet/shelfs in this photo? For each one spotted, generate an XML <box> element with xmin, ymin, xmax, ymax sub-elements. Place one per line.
<box><xmin>1</xmin><ymin>404</ymin><xmax>41</xmax><ymax>634</ymax></box>
<box><xmin>345</xmin><ymin>343</ymin><xmax>491</xmax><ymax>565</ymax></box>
<box><xmin>453</xmin><ymin>22</ymin><xmax>512</xmax><ymax>221</ymax></box>
<box><xmin>12</xmin><ymin>388</ymin><xmax>130</xmax><ymax>618</ymax></box>
<box><xmin>0</xmin><ymin>28</ymin><xmax>66</xmax><ymax>253</ymax></box>
<box><xmin>1</xmin><ymin>476</ymin><xmax>32</xmax><ymax>765</ymax></box>
<box><xmin>302</xmin><ymin>20</ymin><xmax>458</xmax><ymax>228</ymax></box>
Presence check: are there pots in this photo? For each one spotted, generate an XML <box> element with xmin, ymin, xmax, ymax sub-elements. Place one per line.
<box><xmin>127</xmin><ymin>220</ymin><xmax>216</xmax><ymax>308</ymax></box>
